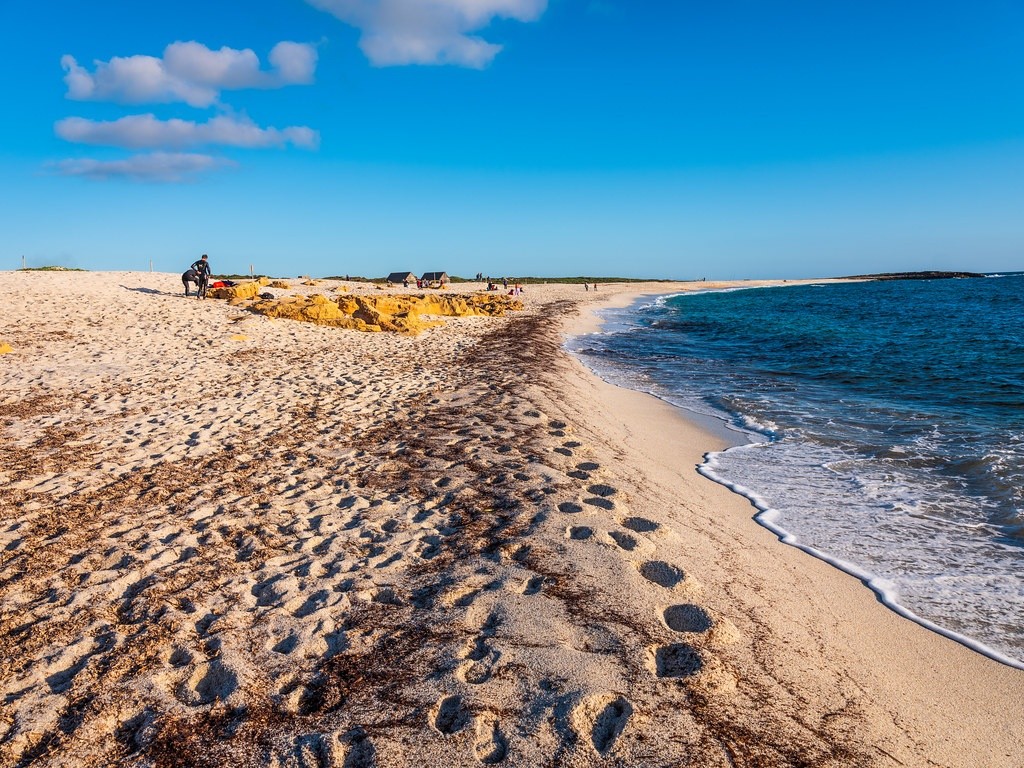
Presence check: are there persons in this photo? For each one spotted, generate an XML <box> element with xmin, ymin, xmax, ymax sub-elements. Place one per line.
<box><xmin>502</xmin><ymin>278</ymin><xmax>508</xmax><ymax>289</ymax></box>
<box><xmin>402</xmin><ymin>277</ymin><xmax>410</xmax><ymax>287</ymax></box>
<box><xmin>584</xmin><ymin>282</ymin><xmax>589</xmax><ymax>291</ymax></box>
<box><xmin>190</xmin><ymin>254</ymin><xmax>211</xmax><ymax>300</ymax></box>
<box><xmin>416</xmin><ymin>277</ymin><xmax>435</xmax><ymax>289</ymax></box>
<box><xmin>520</xmin><ymin>287</ymin><xmax>523</xmax><ymax>291</ymax></box>
<box><xmin>515</xmin><ymin>282</ymin><xmax>519</xmax><ymax>291</ymax></box>
<box><xmin>593</xmin><ymin>283</ymin><xmax>597</xmax><ymax>289</ymax></box>
<box><xmin>439</xmin><ymin>279</ymin><xmax>443</xmax><ymax>288</ymax></box>
<box><xmin>476</xmin><ymin>273</ymin><xmax>499</xmax><ymax>290</ymax></box>
<box><xmin>182</xmin><ymin>269</ymin><xmax>200</xmax><ymax>296</ymax></box>
<box><xmin>507</xmin><ymin>289</ymin><xmax>514</xmax><ymax>295</ymax></box>
